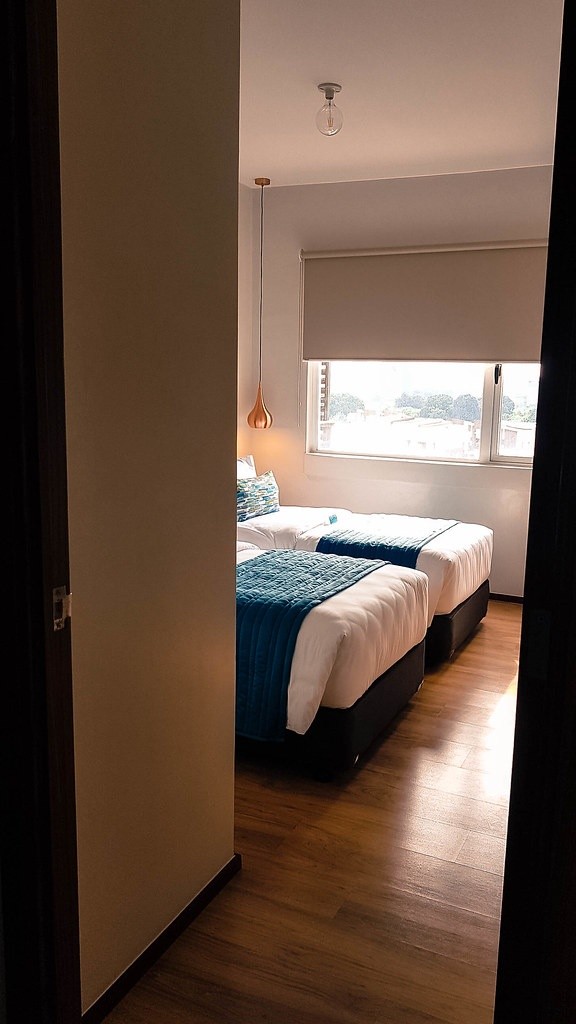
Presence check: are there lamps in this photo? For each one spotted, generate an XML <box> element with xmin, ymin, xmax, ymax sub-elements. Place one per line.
<box><xmin>247</xmin><ymin>178</ymin><xmax>273</xmax><ymax>429</ymax></box>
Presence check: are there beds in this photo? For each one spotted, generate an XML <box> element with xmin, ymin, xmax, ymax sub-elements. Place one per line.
<box><xmin>237</xmin><ymin>504</ymin><xmax>494</xmax><ymax>660</ymax></box>
<box><xmin>236</xmin><ymin>540</ymin><xmax>430</xmax><ymax>778</ymax></box>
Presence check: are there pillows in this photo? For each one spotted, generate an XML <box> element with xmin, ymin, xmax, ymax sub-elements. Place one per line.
<box><xmin>237</xmin><ymin>469</ymin><xmax>280</xmax><ymax>522</ymax></box>
<box><xmin>237</xmin><ymin>454</ymin><xmax>258</xmax><ymax>480</ymax></box>
